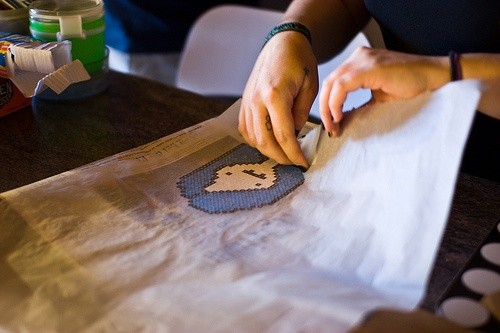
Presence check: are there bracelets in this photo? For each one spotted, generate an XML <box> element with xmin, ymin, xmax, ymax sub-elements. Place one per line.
<box><xmin>449</xmin><ymin>51</ymin><xmax>463</xmax><ymax>82</ymax></box>
<box><xmin>262</xmin><ymin>22</ymin><xmax>312</xmax><ymax>50</ymax></box>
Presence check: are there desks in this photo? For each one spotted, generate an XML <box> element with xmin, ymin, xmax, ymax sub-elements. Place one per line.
<box><xmin>0</xmin><ymin>68</ymin><xmax>500</xmax><ymax>333</ymax></box>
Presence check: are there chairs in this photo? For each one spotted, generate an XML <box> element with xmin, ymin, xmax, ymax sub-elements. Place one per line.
<box><xmin>175</xmin><ymin>5</ymin><xmax>386</xmax><ymax>120</ymax></box>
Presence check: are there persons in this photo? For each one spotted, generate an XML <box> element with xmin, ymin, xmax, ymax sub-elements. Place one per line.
<box><xmin>237</xmin><ymin>0</ymin><xmax>500</xmax><ymax>174</ymax></box>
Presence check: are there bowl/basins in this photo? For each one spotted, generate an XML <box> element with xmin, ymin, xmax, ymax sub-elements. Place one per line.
<box><xmin>0</xmin><ymin>0</ymin><xmax>110</xmax><ymax>100</ymax></box>
